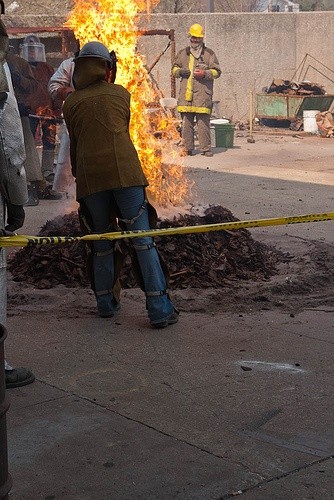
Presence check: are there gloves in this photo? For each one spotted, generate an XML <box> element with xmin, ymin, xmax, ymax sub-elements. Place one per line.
<box><xmin>5</xmin><ymin>204</ymin><xmax>25</xmax><ymax>232</ymax></box>
<box><xmin>181</xmin><ymin>69</ymin><xmax>190</xmax><ymax>78</ymax></box>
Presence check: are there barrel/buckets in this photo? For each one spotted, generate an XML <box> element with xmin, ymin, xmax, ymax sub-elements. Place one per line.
<box><xmin>214</xmin><ymin>124</ymin><xmax>234</xmax><ymax>148</ymax></box>
<box><xmin>302</xmin><ymin>110</ymin><xmax>320</xmax><ymax>132</ymax></box>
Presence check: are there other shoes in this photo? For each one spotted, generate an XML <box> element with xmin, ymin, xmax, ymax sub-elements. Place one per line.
<box><xmin>151</xmin><ymin>307</ymin><xmax>179</xmax><ymax>328</ymax></box>
<box><xmin>26</xmin><ymin>183</ymin><xmax>39</xmax><ymax>206</ymax></box>
<box><xmin>37</xmin><ymin>182</ymin><xmax>62</xmax><ymax>200</ymax></box>
<box><xmin>98</xmin><ymin>302</ymin><xmax>120</xmax><ymax>318</ymax></box>
<box><xmin>4</xmin><ymin>366</ymin><xmax>35</xmax><ymax>388</ymax></box>
<box><xmin>186</xmin><ymin>150</ymin><xmax>192</xmax><ymax>155</ymax></box>
<box><xmin>203</xmin><ymin>151</ymin><xmax>212</xmax><ymax>157</ymax></box>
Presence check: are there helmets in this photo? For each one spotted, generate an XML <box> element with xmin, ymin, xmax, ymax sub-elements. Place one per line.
<box><xmin>78</xmin><ymin>42</ymin><xmax>113</xmax><ymax>64</ymax></box>
<box><xmin>188</xmin><ymin>24</ymin><xmax>204</xmax><ymax>38</ymax></box>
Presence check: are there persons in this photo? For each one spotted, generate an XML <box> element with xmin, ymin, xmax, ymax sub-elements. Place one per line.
<box><xmin>0</xmin><ymin>20</ymin><xmax>35</xmax><ymax>388</ymax></box>
<box><xmin>48</xmin><ymin>50</ymin><xmax>81</xmax><ymax>200</ymax></box>
<box><xmin>63</xmin><ymin>41</ymin><xmax>180</xmax><ymax>329</ymax></box>
<box><xmin>171</xmin><ymin>23</ymin><xmax>221</xmax><ymax>157</ymax></box>
<box><xmin>6</xmin><ymin>38</ymin><xmax>43</xmax><ymax>206</ymax></box>
